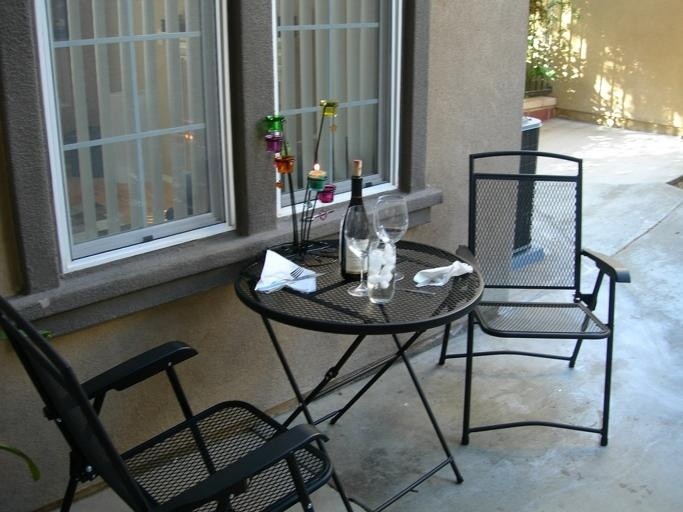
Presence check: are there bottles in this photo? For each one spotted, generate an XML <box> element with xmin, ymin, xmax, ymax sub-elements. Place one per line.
<box><xmin>340</xmin><ymin>158</ymin><xmax>372</xmax><ymax>282</ymax></box>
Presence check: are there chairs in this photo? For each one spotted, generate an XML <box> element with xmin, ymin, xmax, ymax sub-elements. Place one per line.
<box><xmin>439</xmin><ymin>151</ymin><xmax>631</xmax><ymax>449</ymax></box>
<box><xmin>0</xmin><ymin>295</ymin><xmax>335</xmax><ymax>511</ymax></box>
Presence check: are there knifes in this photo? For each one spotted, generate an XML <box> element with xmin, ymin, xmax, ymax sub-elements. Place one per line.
<box><xmin>258</xmin><ymin>272</ymin><xmax>326</xmax><ymax>290</ymax></box>
<box><xmin>415</xmin><ymin>269</ymin><xmax>444</xmax><ymax>288</ymax></box>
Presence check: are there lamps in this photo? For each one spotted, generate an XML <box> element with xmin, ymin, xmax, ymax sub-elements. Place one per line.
<box><xmin>260</xmin><ymin>99</ymin><xmax>338</xmax><ymax>268</ymax></box>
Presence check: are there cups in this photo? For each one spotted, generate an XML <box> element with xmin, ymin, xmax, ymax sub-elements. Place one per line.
<box><xmin>366</xmin><ymin>241</ymin><xmax>395</xmax><ymax>306</ymax></box>
<box><xmin>372</xmin><ymin>195</ymin><xmax>409</xmax><ymax>283</ymax></box>
<box><xmin>344</xmin><ymin>204</ymin><xmax>381</xmax><ymax>298</ymax></box>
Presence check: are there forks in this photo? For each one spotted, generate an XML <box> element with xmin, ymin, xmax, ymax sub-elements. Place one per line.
<box><xmin>261</xmin><ymin>266</ymin><xmax>304</xmax><ymax>293</ymax></box>
